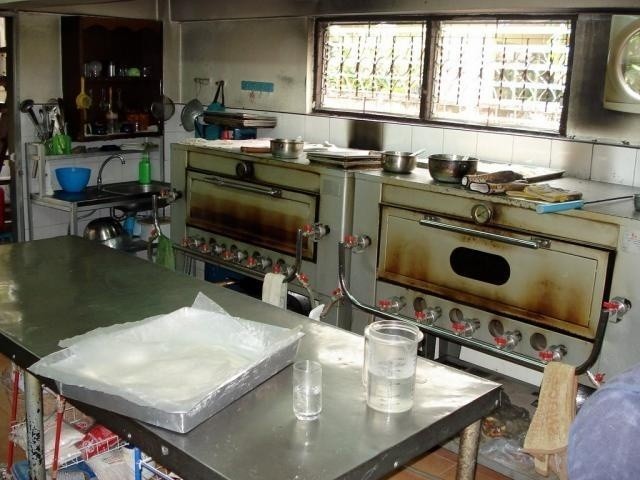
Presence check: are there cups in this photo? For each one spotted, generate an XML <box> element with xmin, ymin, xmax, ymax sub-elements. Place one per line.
<box><xmin>292</xmin><ymin>361</ymin><xmax>322</xmax><ymax>421</ymax></box>
<box><xmin>106</xmin><ymin>114</ymin><xmax>118</xmax><ymax>133</ymax></box>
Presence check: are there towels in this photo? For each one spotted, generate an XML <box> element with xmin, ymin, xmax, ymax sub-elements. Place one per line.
<box><xmin>524</xmin><ymin>361</ymin><xmax>582</xmax><ymax>480</ymax></box>
<box><xmin>261</xmin><ymin>270</ymin><xmax>291</xmax><ymax>312</ymax></box>
<box><xmin>156</xmin><ymin>235</ymin><xmax>176</xmax><ymax>272</ymax></box>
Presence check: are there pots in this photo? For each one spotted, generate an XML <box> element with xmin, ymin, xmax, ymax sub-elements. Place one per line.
<box><xmin>149</xmin><ymin>78</ymin><xmax>175</xmax><ymax>122</ymax></box>
<box><xmin>179</xmin><ymin>99</ymin><xmax>204</xmax><ymax>131</ymax></box>
<box><xmin>270</xmin><ymin>136</ymin><xmax>304</xmax><ymax>159</ymax></box>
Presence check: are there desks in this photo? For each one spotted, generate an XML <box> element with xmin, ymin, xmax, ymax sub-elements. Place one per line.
<box><xmin>0</xmin><ymin>235</ymin><xmax>503</xmax><ymax>480</ymax></box>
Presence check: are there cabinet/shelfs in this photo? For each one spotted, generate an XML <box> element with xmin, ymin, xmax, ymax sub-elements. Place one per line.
<box><xmin>61</xmin><ymin>16</ymin><xmax>164</xmax><ymax>142</ymax></box>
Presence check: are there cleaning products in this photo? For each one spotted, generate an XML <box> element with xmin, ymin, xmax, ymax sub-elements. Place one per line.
<box><xmin>138</xmin><ymin>151</ymin><xmax>151</xmax><ymax>185</ymax></box>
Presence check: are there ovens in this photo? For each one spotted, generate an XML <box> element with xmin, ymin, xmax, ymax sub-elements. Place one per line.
<box><xmin>170</xmin><ymin>138</ymin><xmax>355</xmax><ymax>330</ymax></box>
<box><xmin>338</xmin><ymin>172</ymin><xmax>640</xmax><ymax>390</ymax></box>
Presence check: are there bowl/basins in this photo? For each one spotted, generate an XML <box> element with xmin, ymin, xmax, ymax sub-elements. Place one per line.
<box><xmin>101</xmin><ymin>235</ymin><xmax>129</xmax><ymax>250</ymax></box>
<box><xmin>428</xmin><ymin>154</ymin><xmax>479</xmax><ymax>184</ymax></box>
<box><xmin>83</xmin><ymin>216</ymin><xmax>123</xmax><ymax>243</ymax></box>
<box><xmin>55</xmin><ymin>168</ymin><xmax>91</xmax><ymax>193</ymax></box>
<box><xmin>380</xmin><ymin>146</ymin><xmax>425</xmax><ymax>173</ymax></box>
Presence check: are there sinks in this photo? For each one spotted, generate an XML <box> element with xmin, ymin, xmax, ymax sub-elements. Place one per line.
<box><xmin>104</xmin><ymin>180</ymin><xmax>174</xmax><ymax>195</ymax></box>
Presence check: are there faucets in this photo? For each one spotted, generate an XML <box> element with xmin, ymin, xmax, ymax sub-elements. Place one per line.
<box><xmin>97</xmin><ymin>154</ymin><xmax>126</xmax><ymax>190</ymax></box>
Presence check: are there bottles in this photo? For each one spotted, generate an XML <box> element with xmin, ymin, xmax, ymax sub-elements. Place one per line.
<box><xmin>120</xmin><ymin>65</ymin><xmax>123</xmax><ymax>76</ymax></box>
<box><xmin>109</xmin><ymin>59</ymin><xmax>115</xmax><ymax>77</ymax></box>
<box><xmin>125</xmin><ymin>65</ymin><xmax>128</xmax><ymax>75</ymax></box>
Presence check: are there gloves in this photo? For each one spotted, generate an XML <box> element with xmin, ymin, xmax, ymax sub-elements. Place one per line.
<box><xmin>461</xmin><ymin>171</ymin><xmax>522</xmax><ymax>188</ymax></box>
<box><xmin>469</xmin><ymin>179</ymin><xmax>528</xmax><ymax>194</ymax></box>
